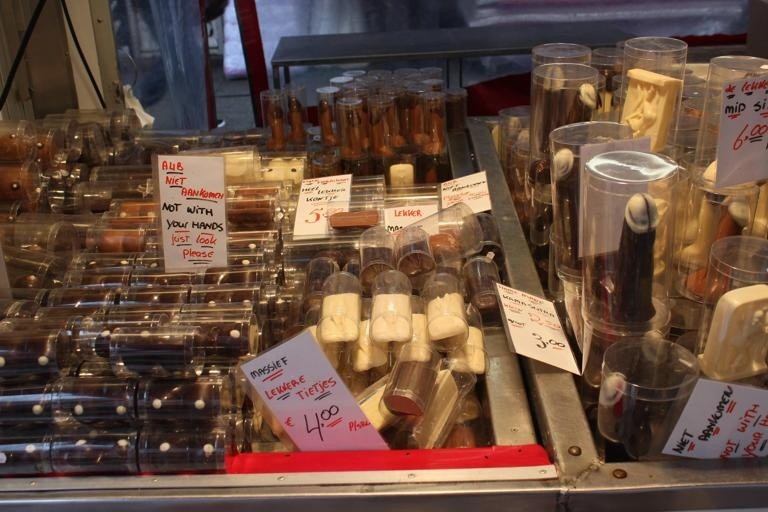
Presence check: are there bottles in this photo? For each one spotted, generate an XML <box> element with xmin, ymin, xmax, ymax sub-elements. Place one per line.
<box><xmin>0</xmin><ymin>36</ymin><xmax>767</xmax><ymax>474</ymax></box>
